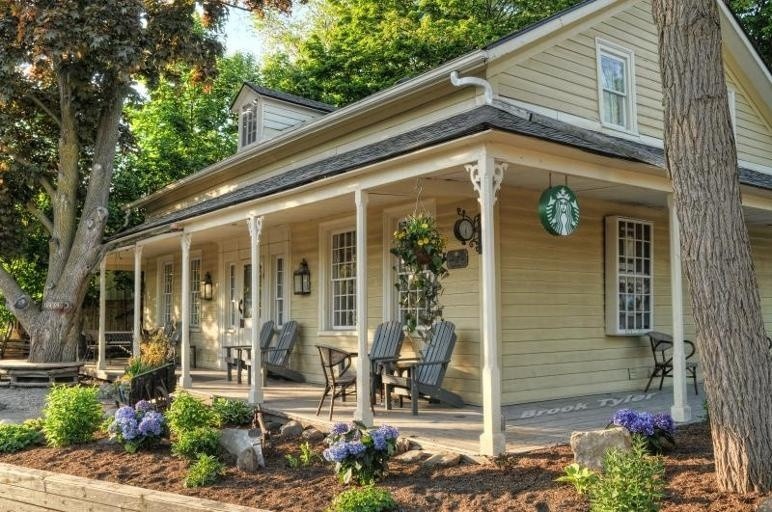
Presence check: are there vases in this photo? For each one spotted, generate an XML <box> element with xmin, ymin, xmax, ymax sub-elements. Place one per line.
<box><xmin>416</xmin><ymin>252</ymin><xmax>431</xmax><ymax>264</ymax></box>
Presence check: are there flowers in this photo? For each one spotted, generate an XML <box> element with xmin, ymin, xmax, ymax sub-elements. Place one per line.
<box><xmin>390</xmin><ymin>206</ymin><xmax>450</xmax><ymax>334</ymax></box>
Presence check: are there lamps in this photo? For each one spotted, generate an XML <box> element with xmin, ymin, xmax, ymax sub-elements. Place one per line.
<box><xmin>199</xmin><ymin>272</ymin><xmax>212</xmax><ymax>300</ymax></box>
<box><xmin>294</xmin><ymin>257</ymin><xmax>310</xmax><ymax>295</ymax></box>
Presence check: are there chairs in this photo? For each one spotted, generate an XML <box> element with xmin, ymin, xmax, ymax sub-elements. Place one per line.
<box><xmin>222</xmin><ymin>321</ymin><xmax>465</xmax><ymax>421</ymax></box>
<box><xmin>645</xmin><ymin>331</ymin><xmax>698</xmax><ymax>395</ymax></box>
<box><xmin>80</xmin><ymin>330</ymin><xmax>112</xmax><ymax>367</ymax></box>
